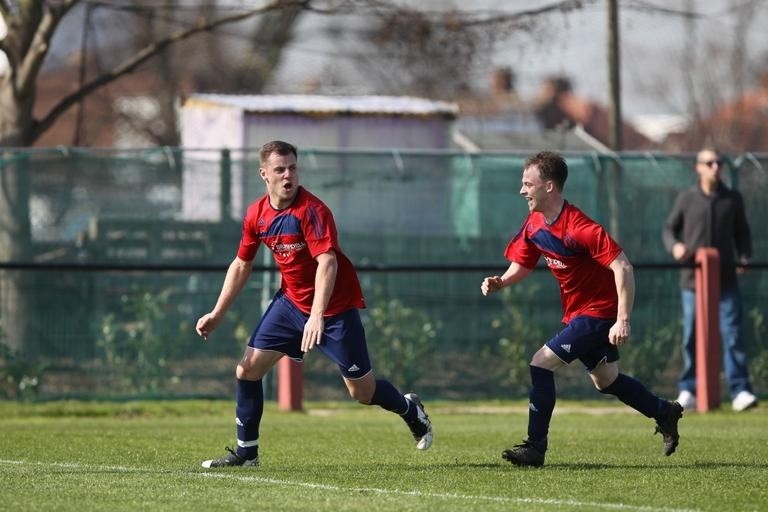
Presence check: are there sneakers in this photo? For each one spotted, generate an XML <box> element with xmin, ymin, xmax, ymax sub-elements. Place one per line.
<box><xmin>201</xmin><ymin>446</ymin><xmax>260</xmax><ymax>468</ymax></box>
<box><xmin>405</xmin><ymin>393</ymin><xmax>434</xmax><ymax>450</ymax></box>
<box><xmin>677</xmin><ymin>390</ymin><xmax>697</xmax><ymax>410</ymax></box>
<box><xmin>503</xmin><ymin>440</ymin><xmax>545</xmax><ymax>467</ymax></box>
<box><xmin>655</xmin><ymin>401</ymin><xmax>684</xmax><ymax>456</ymax></box>
<box><xmin>732</xmin><ymin>391</ymin><xmax>758</xmax><ymax>412</ymax></box>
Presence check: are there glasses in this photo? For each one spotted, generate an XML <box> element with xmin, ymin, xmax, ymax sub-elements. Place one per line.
<box><xmin>702</xmin><ymin>160</ymin><xmax>722</xmax><ymax>167</ymax></box>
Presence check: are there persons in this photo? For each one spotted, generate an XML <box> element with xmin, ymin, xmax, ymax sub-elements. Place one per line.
<box><xmin>660</xmin><ymin>143</ymin><xmax>759</xmax><ymax>415</ymax></box>
<box><xmin>194</xmin><ymin>141</ymin><xmax>433</xmax><ymax>469</ymax></box>
<box><xmin>480</xmin><ymin>152</ymin><xmax>684</xmax><ymax>470</ymax></box>
